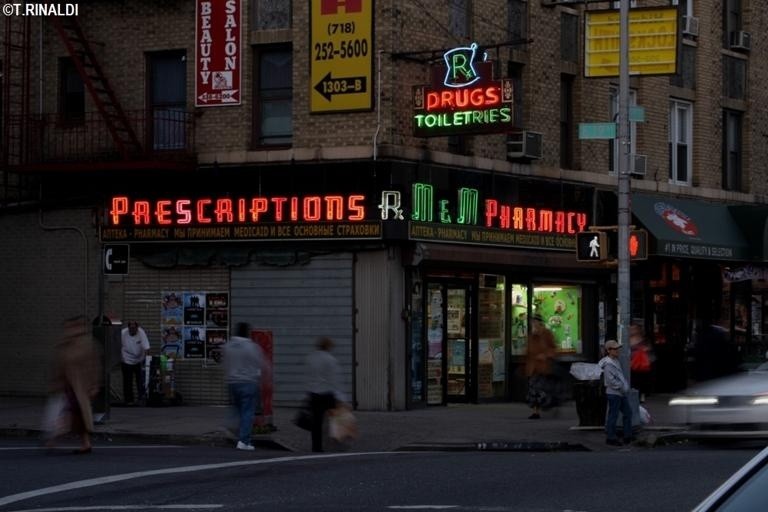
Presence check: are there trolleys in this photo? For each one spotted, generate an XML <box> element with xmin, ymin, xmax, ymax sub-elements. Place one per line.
<box><xmin>147</xmin><ymin>343</ymin><xmax>183</xmax><ymax>408</ymax></box>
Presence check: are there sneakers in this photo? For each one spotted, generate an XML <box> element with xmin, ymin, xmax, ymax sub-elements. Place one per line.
<box><xmin>606</xmin><ymin>438</ymin><xmax>622</xmax><ymax>446</ymax></box>
<box><xmin>623</xmin><ymin>436</ymin><xmax>636</xmax><ymax>445</ymax></box>
<box><xmin>235</xmin><ymin>440</ymin><xmax>255</xmax><ymax>450</ymax></box>
<box><xmin>527</xmin><ymin>413</ymin><xmax>540</xmax><ymax>419</ymax></box>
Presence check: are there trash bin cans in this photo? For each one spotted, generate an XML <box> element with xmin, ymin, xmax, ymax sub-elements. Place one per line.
<box><xmin>570</xmin><ymin>362</ymin><xmax>608</xmax><ymax>426</ymax></box>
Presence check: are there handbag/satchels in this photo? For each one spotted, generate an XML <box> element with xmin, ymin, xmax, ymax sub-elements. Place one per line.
<box><xmin>291</xmin><ymin>406</ymin><xmax>314</xmax><ymax>431</ymax></box>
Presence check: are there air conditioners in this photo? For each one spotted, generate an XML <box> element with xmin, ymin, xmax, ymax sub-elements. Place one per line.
<box><xmin>731</xmin><ymin>30</ymin><xmax>752</xmax><ymax>51</ymax></box>
<box><xmin>507</xmin><ymin>129</ymin><xmax>545</xmax><ymax>161</ymax></box>
<box><xmin>629</xmin><ymin>153</ymin><xmax>648</xmax><ymax>176</ymax></box>
<box><xmin>682</xmin><ymin>15</ymin><xmax>700</xmax><ymax>36</ymax></box>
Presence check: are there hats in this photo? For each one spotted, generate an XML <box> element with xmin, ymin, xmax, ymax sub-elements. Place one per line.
<box><xmin>605</xmin><ymin>340</ymin><xmax>622</xmax><ymax>350</ymax></box>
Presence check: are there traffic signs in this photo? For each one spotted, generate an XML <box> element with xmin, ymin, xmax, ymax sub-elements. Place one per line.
<box><xmin>628</xmin><ymin>107</ymin><xmax>646</xmax><ymax>123</ymax></box>
<box><xmin>579</xmin><ymin>123</ymin><xmax>617</xmax><ymax>141</ymax></box>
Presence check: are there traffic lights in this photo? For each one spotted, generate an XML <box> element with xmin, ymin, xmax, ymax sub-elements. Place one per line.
<box><xmin>574</xmin><ymin>230</ymin><xmax>611</xmax><ymax>263</ymax></box>
<box><xmin>624</xmin><ymin>229</ymin><xmax>649</xmax><ymax>261</ymax></box>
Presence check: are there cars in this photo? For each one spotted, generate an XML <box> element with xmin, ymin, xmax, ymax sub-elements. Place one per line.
<box><xmin>693</xmin><ymin>427</ymin><xmax>767</xmax><ymax>512</ymax></box>
<box><xmin>664</xmin><ymin>353</ymin><xmax>767</xmax><ymax>427</ymax></box>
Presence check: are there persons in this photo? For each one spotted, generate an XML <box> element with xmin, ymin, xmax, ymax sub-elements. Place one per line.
<box><xmin>40</xmin><ymin>330</ymin><xmax>103</xmax><ymax>456</ymax></box>
<box><xmin>223</xmin><ymin>322</ymin><xmax>264</xmax><ymax>451</ymax></box>
<box><xmin>304</xmin><ymin>337</ymin><xmax>346</xmax><ymax>452</ymax></box>
<box><xmin>525</xmin><ymin>314</ymin><xmax>558</xmax><ymax>420</ymax></box>
<box><xmin>630</xmin><ymin>323</ymin><xmax>653</xmax><ymax>404</ymax></box>
<box><xmin>598</xmin><ymin>339</ymin><xmax>637</xmax><ymax>448</ymax></box>
<box><xmin>120</xmin><ymin>319</ymin><xmax>150</xmax><ymax>407</ymax></box>
